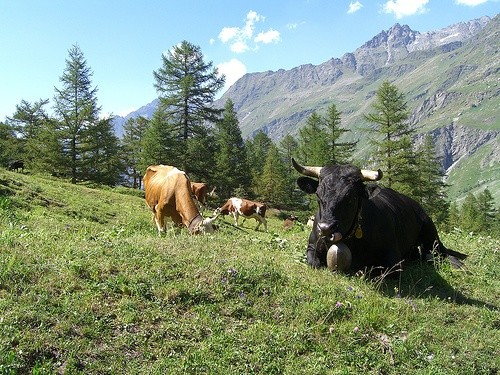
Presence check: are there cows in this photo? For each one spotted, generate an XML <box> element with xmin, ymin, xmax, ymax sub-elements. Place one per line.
<box><xmin>290</xmin><ymin>157</ymin><xmax>469</xmax><ymax>287</ymax></box>
<box><xmin>216</xmin><ymin>196</ymin><xmax>268</xmax><ymax>233</ymax></box>
<box><xmin>282</xmin><ymin>214</ymin><xmax>296</xmax><ymax>229</ymax></box>
<box><xmin>7</xmin><ymin>160</ymin><xmax>24</xmax><ymax>174</ymax></box>
<box><xmin>142</xmin><ymin>164</ymin><xmax>222</xmax><ymax>237</ymax></box>
<box><xmin>51</xmin><ymin>172</ymin><xmax>66</xmax><ymax>179</ymax></box>
